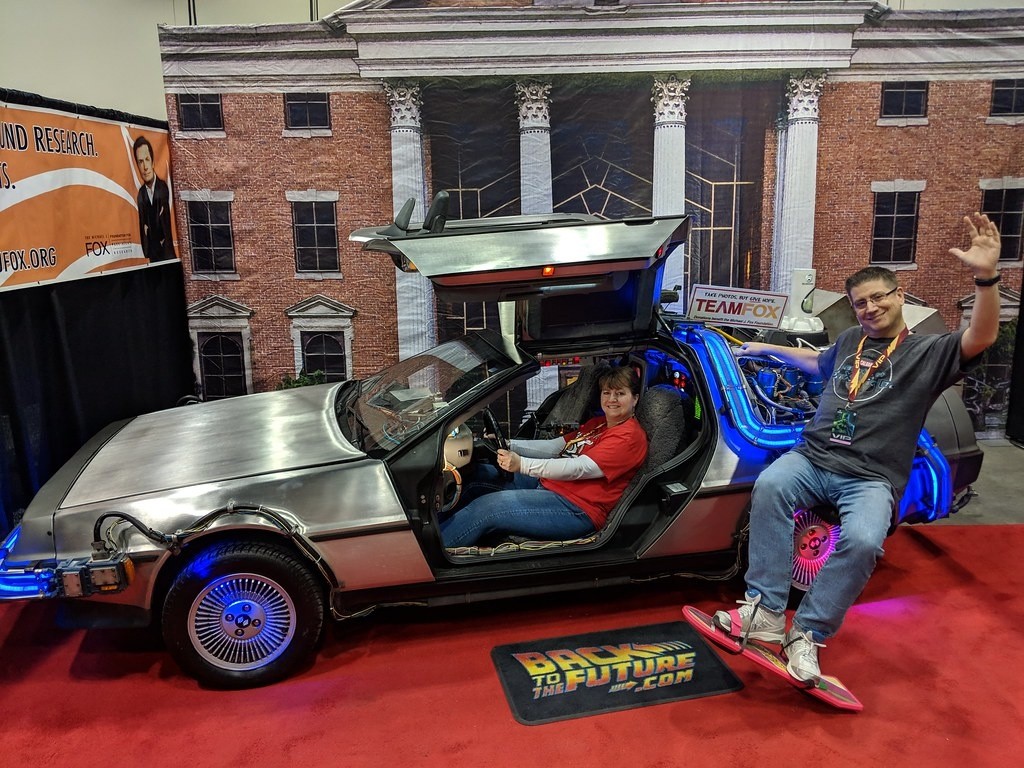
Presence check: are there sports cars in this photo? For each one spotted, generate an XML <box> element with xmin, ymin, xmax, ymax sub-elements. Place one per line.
<box><xmin>1</xmin><ymin>187</ymin><xmax>986</xmax><ymax>691</ymax></box>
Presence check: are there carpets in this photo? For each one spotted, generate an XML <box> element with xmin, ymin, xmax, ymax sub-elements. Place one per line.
<box><xmin>489</xmin><ymin>621</ymin><xmax>745</xmax><ymax>726</ymax></box>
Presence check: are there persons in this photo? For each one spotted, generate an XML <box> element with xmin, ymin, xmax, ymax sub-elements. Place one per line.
<box><xmin>441</xmin><ymin>367</ymin><xmax>649</xmax><ymax>549</ymax></box>
<box><xmin>134</xmin><ymin>134</ymin><xmax>176</xmax><ymax>264</ymax></box>
<box><xmin>713</xmin><ymin>211</ymin><xmax>1001</xmax><ymax>687</ymax></box>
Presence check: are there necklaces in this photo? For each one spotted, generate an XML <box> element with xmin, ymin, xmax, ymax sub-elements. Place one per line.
<box><xmin>563</xmin><ymin>423</ymin><xmax>605</xmax><ymax>454</ymax></box>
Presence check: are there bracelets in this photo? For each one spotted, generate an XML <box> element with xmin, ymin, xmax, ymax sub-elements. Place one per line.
<box><xmin>974</xmin><ymin>271</ymin><xmax>1002</xmax><ymax>286</ymax></box>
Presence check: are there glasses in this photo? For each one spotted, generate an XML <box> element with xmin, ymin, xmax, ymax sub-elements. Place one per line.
<box><xmin>852</xmin><ymin>286</ymin><xmax>898</xmax><ymax>309</ymax></box>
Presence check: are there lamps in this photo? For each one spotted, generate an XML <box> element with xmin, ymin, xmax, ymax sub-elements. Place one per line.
<box><xmin>320</xmin><ymin>14</ymin><xmax>346</xmax><ymax>39</ymax></box>
<box><xmin>864</xmin><ymin>3</ymin><xmax>894</xmax><ymax>28</ymax></box>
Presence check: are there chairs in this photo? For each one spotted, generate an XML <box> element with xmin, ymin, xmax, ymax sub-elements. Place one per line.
<box><xmin>501</xmin><ymin>383</ymin><xmax>695</xmax><ymax>544</ymax></box>
<box><xmin>513</xmin><ymin>362</ymin><xmax>613</xmax><ymax>439</ymax></box>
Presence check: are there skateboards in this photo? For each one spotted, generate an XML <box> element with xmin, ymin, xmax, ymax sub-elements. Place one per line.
<box><xmin>682</xmin><ymin>605</ymin><xmax>865</xmax><ymax>713</ymax></box>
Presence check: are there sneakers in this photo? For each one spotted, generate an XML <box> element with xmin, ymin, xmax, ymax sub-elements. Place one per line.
<box><xmin>713</xmin><ymin>589</ymin><xmax>786</xmax><ymax>645</ymax></box>
<box><xmin>782</xmin><ymin>625</ymin><xmax>827</xmax><ymax>685</ymax></box>
